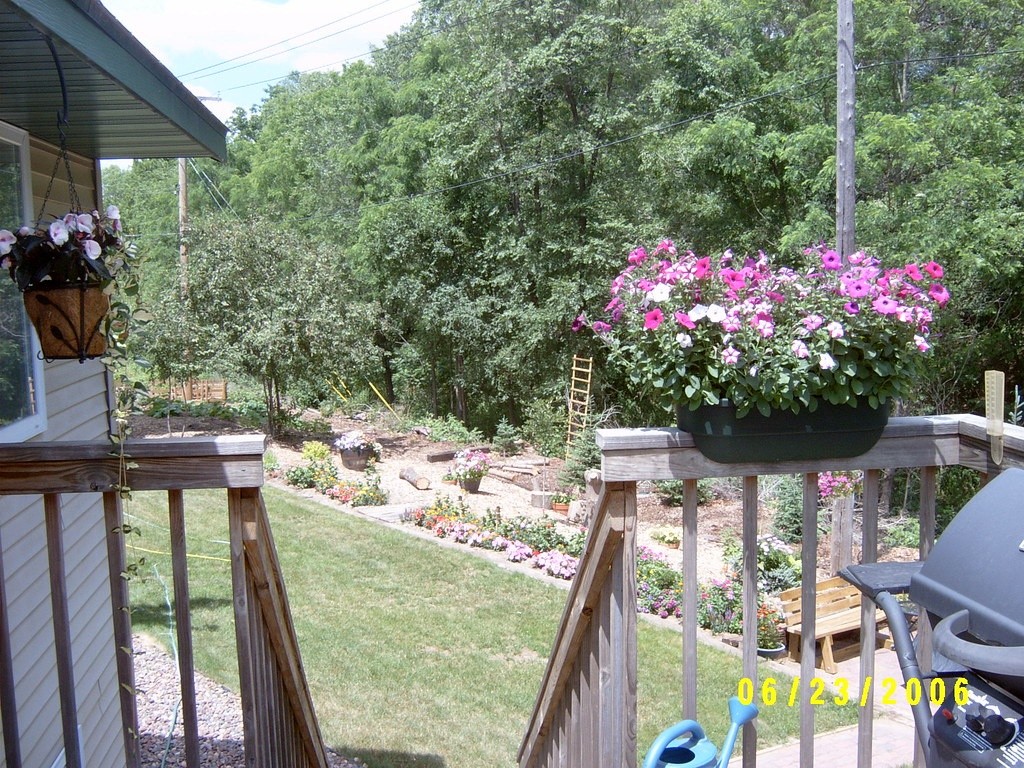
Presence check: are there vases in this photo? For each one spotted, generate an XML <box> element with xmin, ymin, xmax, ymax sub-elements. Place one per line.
<box><xmin>757</xmin><ymin>642</ymin><xmax>786</xmax><ymax>660</ymax></box>
<box><xmin>552</xmin><ymin>504</ymin><xmax>569</xmax><ymax>515</ymax></box>
<box><xmin>457</xmin><ymin>477</ymin><xmax>482</xmax><ymax>493</ymax></box>
<box><xmin>676</xmin><ymin>392</ymin><xmax>888</xmax><ymax>463</ymax></box>
<box><xmin>341</xmin><ymin>450</ymin><xmax>370</xmax><ymax>471</ymax></box>
<box><xmin>661</xmin><ymin>542</ymin><xmax>680</xmax><ymax>549</ymax></box>
<box><xmin>22</xmin><ymin>280</ymin><xmax>112</xmax><ymax>360</ymax></box>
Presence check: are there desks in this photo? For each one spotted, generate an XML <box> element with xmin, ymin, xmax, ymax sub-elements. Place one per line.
<box><xmin>891</xmin><ymin>600</ymin><xmax>921</xmax><ymax>650</ymax></box>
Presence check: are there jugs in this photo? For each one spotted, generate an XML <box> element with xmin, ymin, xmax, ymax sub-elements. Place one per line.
<box><xmin>643</xmin><ymin>697</ymin><xmax>759</xmax><ymax>768</ymax></box>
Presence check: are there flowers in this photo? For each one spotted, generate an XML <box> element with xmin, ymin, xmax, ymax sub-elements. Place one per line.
<box><xmin>756</xmin><ymin>603</ymin><xmax>783</xmax><ymax>650</ymax></box>
<box><xmin>0</xmin><ymin>206</ymin><xmax>148</xmax><ymax>586</ymax></box>
<box><xmin>551</xmin><ymin>486</ymin><xmax>573</xmax><ymax>504</ymax></box>
<box><xmin>447</xmin><ymin>448</ymin><xmax>490</xmax><ymax>482</ymax></box>
<box><xmin>572</xmin><ymin>238</ymin><xmax>950</xmax><ymax>419</ymax></box>
<box><xmin>333</xmin><ymin>428</ymin><xmax>374</xmax><ymax>457</ymax></box>
<box><xmin>650</xmin><ymin>524</ymin><xmax>684</xmax><ymax>543</ymax></box>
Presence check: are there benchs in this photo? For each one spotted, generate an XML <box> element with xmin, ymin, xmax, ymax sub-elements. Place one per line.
<box><xmin>780</xmin><ymin>575</ymin><xmax>888</xmax><ymax>675</ymax></box>
